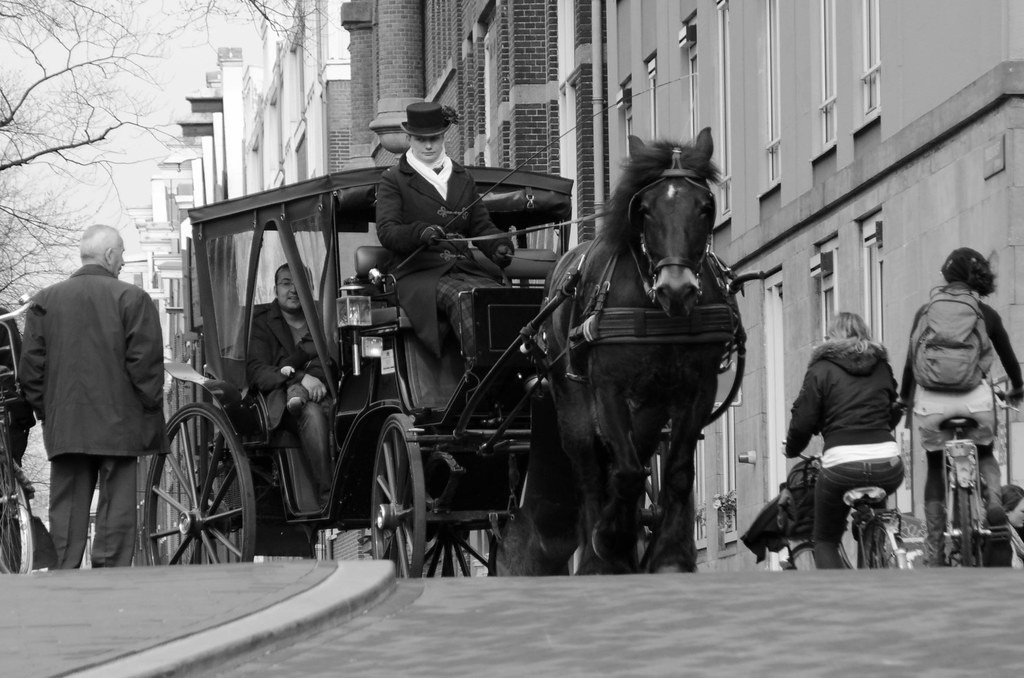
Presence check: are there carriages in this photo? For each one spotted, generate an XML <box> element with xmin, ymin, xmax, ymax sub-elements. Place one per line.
<box><xmin>133</xmin><ymin>127</ymin><xmax>768</xmax><ymax>579</ymax></box>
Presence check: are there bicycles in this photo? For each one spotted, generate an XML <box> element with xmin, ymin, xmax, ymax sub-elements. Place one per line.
<box><xmin>0</xmin><ymin>293</ymin><xmax>33</xmax><ymax>576</ymax></box>
<box><xmin>892</xmin><ymin>392</ymin><xmax>1024</xmax><ymax>569</ymax></box>
<box><xmin>784</xmin><ymin>452</ymin><xmax>908</xmax><ymax>572</ymax></box>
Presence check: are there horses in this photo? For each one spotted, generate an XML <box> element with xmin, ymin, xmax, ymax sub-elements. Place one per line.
<box><xmin>528</xmin><ymin>126</ymin><xmax>731</xmax><ymax>576</ymax></box>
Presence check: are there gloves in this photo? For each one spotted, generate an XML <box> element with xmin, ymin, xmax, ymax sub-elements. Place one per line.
<box><xmin>420</xmin><ymin>224</ymin><xmax>446</xmax><ymax>247</ymax></box>
<box><xmin>492</xmin><ymin>244</ymin><xmax>512</xmax><ymax>268</ymax></box>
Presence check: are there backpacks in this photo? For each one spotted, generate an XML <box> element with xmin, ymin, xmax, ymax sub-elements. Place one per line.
<box><xmin>909</xmin><ymin>277</ymin><xmax>996</xmax><ymax>392</ymax></box>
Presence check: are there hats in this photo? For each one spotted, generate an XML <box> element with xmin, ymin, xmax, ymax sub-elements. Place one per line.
<box><xmin>398</xmin><ymin>101</ymin><xmax>457</xmax><ymax>137</ymax></box>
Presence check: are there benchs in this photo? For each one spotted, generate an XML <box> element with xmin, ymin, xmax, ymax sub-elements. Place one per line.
<box><xmin>342</xmin><ymin>247</ymin><xmax>559</xmax><ymax>327</ymax></box>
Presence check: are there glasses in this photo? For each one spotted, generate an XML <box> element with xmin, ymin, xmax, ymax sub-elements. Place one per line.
<box><xmin>275</xmin><ymin>279</ymin><xmax>292</xmax><ymax>289</ymax></box>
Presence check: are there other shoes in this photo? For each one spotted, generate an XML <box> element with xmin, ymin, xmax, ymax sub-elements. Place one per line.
<box><xmin>987</xmin><ymin>503</ymin><xmax>1013</xmax><ymax>544</ymax></box>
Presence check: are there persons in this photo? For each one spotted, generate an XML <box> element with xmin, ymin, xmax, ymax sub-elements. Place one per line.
<box><xmin>0</xmin><ymin>308</ymin><xmax>57</xmax><ymax>571</ymax></box>
<box><xmin>18</xmin><ymin>224</ymin><xmax>165</xmax><ymax>570</ymax></box>
<box><xmin>377</xmin><ymin>101</ymin><xmax>514</xmax><ymax>364</ymax></box>
<box><xmin>246</xmin><ymin>264</ymin><xmax>341</xmax><ymax>511</ymax></box>
<box><xmin>896</xmin><ymin>248</ymin><xmax>1024</xmax><ymax>568</ymax></box>
<box><xmin>777</xmin><ymin>313</ymin><xmax>904</xmax><ymax>569</ymax></box>
